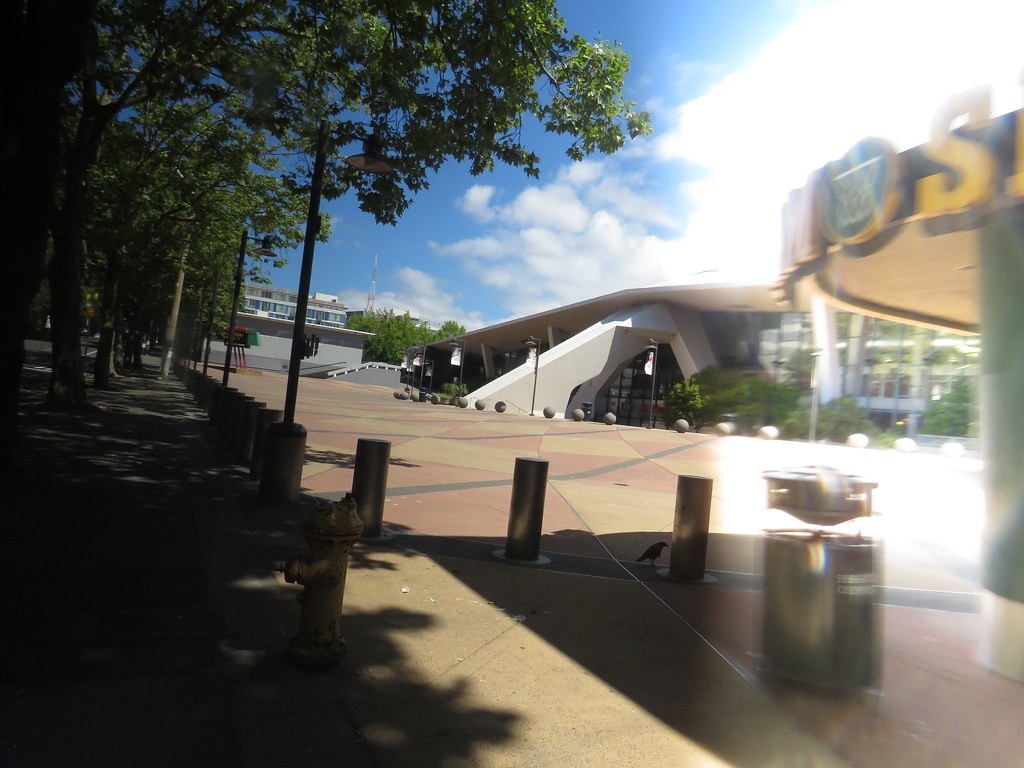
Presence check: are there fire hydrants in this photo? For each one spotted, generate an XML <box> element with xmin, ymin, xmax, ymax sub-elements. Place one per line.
<box><xmin>281</xmin><ymin>496</ymin><xmax>365</xmax><ymax>665</ymax></box>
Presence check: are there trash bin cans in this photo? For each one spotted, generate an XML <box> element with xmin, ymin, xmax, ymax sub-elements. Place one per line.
<box><xmin>759</xmin><ymin>460</ymin><xmax>883</xmax><ymax>696</ymax></box>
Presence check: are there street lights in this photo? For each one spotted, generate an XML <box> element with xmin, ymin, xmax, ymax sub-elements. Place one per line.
<box><xmin>644</xmin><ymin>339</ymin><xmax>658</xmax><ymax>429</ymax></box>
<box><xmin>809</xmin><ymin>352</ymin><xmax>821</xmax><ymax>442</ymax></box>
<box><xmin>203</xmin><ymin>273</ymin><xmax>246</xmax><ymax>375</ymax></box>
<box><xmin>447</xmin><ymin>338</ymin><xmax>465</xmax><ymax>397</ymax></box>
<box><xmin>412</xmin><ymin>341</ymin><xmax>427</xmax><ymax>393</ymax></box>
<box><xmin>524</xmin><ymin>336</ymin><xmax>541</xmax><ymax>416</ymax></box>
<box><xmin>425</xmin><ymin>359</ymin><xmax>434</xmax><ymax>395</ymax></box>
<box><xmin>221</xmin><ymin>233</ymin><xmax>278</xmax><ymax>385</ymax></box>
<box><xmin>258</xmin><ymin>128</ymin><xmax>396</xmax><ymax>511</ymax></box>
